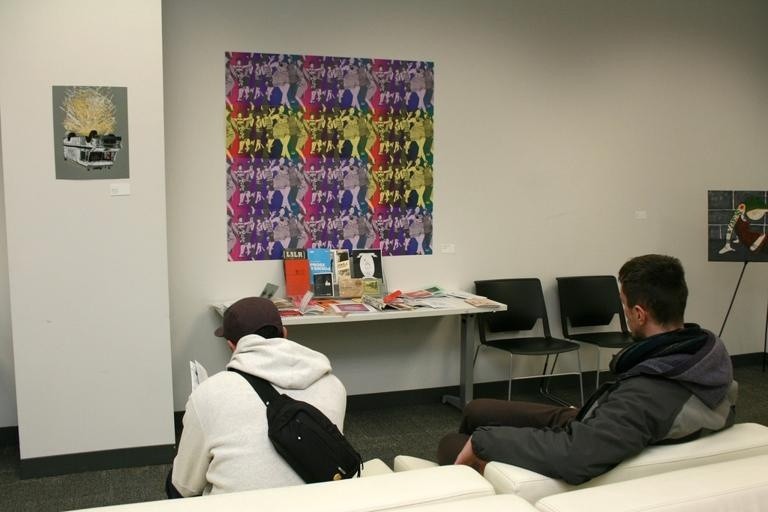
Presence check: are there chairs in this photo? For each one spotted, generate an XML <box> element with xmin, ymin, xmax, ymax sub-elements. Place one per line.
<box><xmin>401</xmin><ymin>493</ymin><xmax>539</xmax><ymax>511</ymax></box>
<box><xmin>393</xmin><ymin>422</ymin><xmax>767</xmax><ymax>503</ymax></box>
<box><xmin>535</xmin><ymin>452</ymin><xmax>767</xmax><ymax>511</ymax></box>
<box><xmin>58</xmin><ymin>457</ymin><xmax>497</xmax><ymax>509</ymax></box>
<box><xmin>467</xmin><ymin>274</ymin><xmax>644</xmax><ymax>412</ymax></box>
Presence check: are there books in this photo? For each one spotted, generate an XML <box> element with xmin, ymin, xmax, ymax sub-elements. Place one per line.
<box><xmin>281</xmin><ymin>249</ymin><xmax>384</xmax><ymax>299</ymax></box>
<box><xmin>463</xmin><ymin>297</ymin><xmax>501</xmax><ymax>308</ymax></box>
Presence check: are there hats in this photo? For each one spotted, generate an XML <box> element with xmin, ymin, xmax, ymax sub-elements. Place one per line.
<box><xmin>214</xmin><ymin>298</ymin><xmax>282</xmax><ymax>343</ymax></box>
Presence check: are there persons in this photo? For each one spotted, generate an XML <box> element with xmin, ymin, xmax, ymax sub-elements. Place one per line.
<box><xmin>437</xmin><ymin>254</ymin><xmax>739</xmax><ymax>487</ymax></box>
<box><xmin>164</xmin><ymin>296</ymin><xmax>347</xmax><ymax>501</ymax></box>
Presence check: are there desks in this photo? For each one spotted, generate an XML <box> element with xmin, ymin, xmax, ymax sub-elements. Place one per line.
<box><xmin>212</xmin><ymin>292</ymin><xmax>509</xmax><ymax>416</ymax></box>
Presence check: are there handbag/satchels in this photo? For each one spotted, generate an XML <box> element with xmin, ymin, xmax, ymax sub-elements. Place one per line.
<box><xmin>266</xmin><ymin>392</ymin><xmax>363</xmax><ymax>483</ymax></box>
